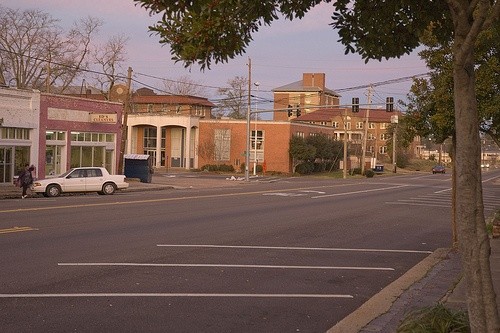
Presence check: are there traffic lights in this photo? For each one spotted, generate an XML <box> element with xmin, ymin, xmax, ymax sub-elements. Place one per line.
<box><xmin>296</xmin><ymin>105</ymin><xmax>301</xmax><ymax>117</ymax></box>
<box><xmin>287</xmin><ymin>104</ymin><xmax>292</xmax><ymax>117</ymax></box>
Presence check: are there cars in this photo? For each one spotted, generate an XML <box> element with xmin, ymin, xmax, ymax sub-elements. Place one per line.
<box><xmin>432</xmin><ymin>166</ymin><xmax>446</xmax><ymax>174</ymax></box>
<box><xmin>30</xmin><ymin>166</ymin><xmax>129</xmax><ymax>197</ymax></box>
<box><xmin>480</xmin><ymin>163</ymin><xmax>490</xmax><ymax>168</ymax></box>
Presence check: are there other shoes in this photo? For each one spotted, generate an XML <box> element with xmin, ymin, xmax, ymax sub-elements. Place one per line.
<box><xmin>22</xmin><ymin>195</ymin><xmax>28</xmax><ymax>199</ymax></box>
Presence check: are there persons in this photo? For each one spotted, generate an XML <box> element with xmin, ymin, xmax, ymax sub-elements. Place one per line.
<box><xmin>18</xmin><ymin>163</ymin><xmax>33</xmax><ymax>199</ymax></box>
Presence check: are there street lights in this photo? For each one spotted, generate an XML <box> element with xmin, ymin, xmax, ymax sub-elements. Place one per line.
<box><xmin>253</xmin><ymin>81</ymin><xmax>260</xmax><ymax>176</ymax></box>
